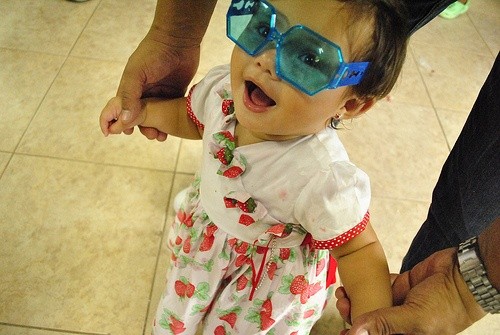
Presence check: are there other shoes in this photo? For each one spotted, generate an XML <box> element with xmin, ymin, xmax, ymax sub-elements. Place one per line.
<box><xmin>439</xmin><ymin>0</ymin><xmax>471</xmax><ymax>19</ymax></box>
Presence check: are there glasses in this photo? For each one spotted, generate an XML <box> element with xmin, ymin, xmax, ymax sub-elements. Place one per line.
<box><xmin>226</xmin><ymin>0</ymin><xmax>373</xmax><ymax>97</ymax></box>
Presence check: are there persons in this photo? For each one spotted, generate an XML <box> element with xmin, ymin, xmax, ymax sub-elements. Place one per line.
<box><xmin>98</xmin><ymin>0</ymin><xmax>412</xmax><ymax>335</ymax></box>
<box><xmin>438</xmin><ymin>0</ymin><xmax>471</xmax><ymax>19</ymax></box>
<box><xmin>117</xmin><ymin>0</ymin><xmax>500</xmax><ymax>335</ymax></box>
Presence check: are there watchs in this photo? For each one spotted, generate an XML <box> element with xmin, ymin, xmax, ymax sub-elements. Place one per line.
<box><xmin>457</xmin><ymin>236</ymin><xmax>500</xmax><ymax>317</ymax></box>
